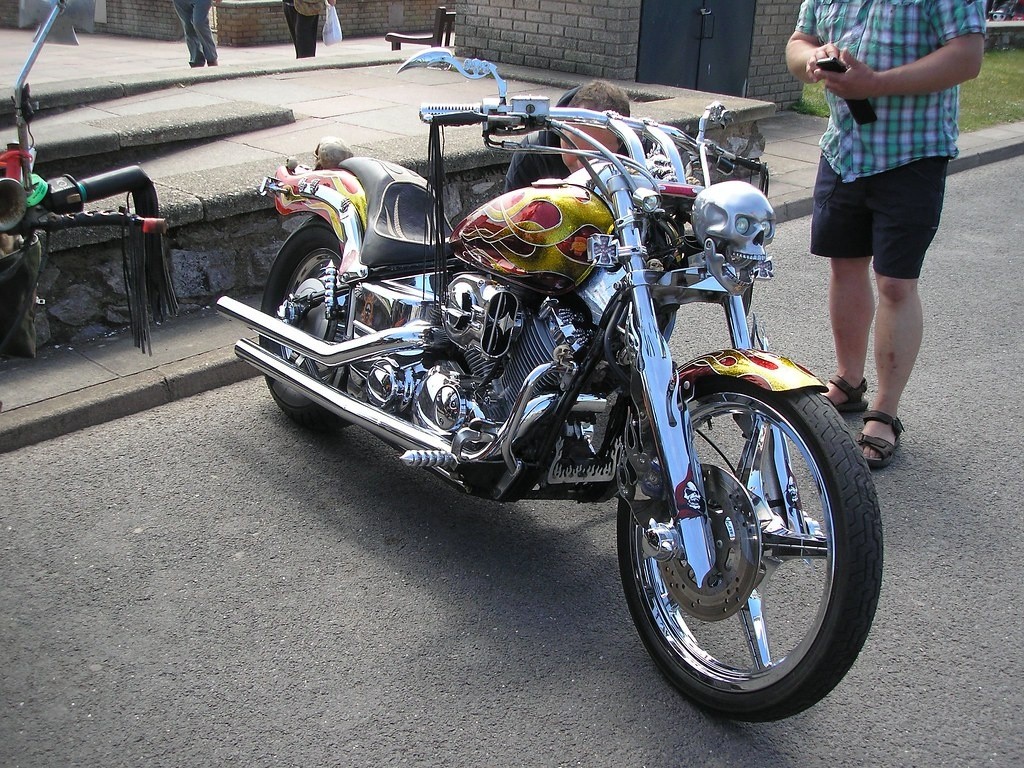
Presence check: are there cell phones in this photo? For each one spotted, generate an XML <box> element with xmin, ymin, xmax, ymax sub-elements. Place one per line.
<box><xmin>816</xmin><ymin>58</ymin><xmax>846</xmax><ymax>73</ymax></box>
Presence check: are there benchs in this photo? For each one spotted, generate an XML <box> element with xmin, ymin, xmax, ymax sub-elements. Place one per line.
<box><xmin>385</xmin><ymin>7</ymin><xmax>456</xmax><ymax>50</ymax></box>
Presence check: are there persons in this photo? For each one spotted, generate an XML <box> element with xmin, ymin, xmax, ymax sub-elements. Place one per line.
<box><xmin>173</xmin><ymin>0</ymin><xmax>223</xmax><ymax>68</ymax></box>
<box><xmin>783</xmin><ymin>0</ymin><xmax>988</xmax><ymax>470</ymax></box>
<box><xmin>501</xmin><ymin>81</ymin><xmax>676</xmax><ymax>395</ymax></box>
<box><xmin>281</xmin><ymin>0</ymin><xmax>336</xmax><ymax>60</ymax></box>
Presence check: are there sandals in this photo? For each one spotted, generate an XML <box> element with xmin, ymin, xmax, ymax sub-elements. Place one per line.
<box><xmin>857</xmin><ymin>413</ymin><xmax>904</xmax><ymax>467</ymax></box>
<box><xmin>821</xmin><ymin>376</ymin><xmax>870</xmax><ymax>413</ymax></box>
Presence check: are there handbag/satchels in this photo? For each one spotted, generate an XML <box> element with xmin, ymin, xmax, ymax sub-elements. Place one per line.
<box><xmin>321</xmin><ymin>1</ymin><xmax>344</xmax><ymax>46</ymax></box>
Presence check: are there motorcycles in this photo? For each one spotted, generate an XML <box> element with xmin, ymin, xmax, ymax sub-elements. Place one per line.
<box><xmin>215</xmin><ymin>50</ymin><xmax>884</xmax><ymax>721</ymax></box>
